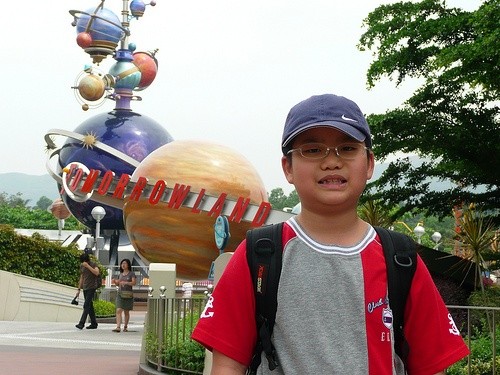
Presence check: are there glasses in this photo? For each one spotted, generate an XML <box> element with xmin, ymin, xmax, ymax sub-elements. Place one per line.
<box><xmin>287</xmin><ymin>143</ymin><xmax>369</xmax><ymax>160</ymax></box>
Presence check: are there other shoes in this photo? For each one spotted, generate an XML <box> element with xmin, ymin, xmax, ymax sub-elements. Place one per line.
<box><xmin>76</xmin><ymin>323</ymin><xmax>83</xmax><ymax>329</ymax></box>
<box><xmin>86</xmin><ymin>325</ymin><xmax>97</xmax><ymax>329</ymax></box>
<box><xmin>123</xmin><ymin>330</ymin><xmax>128</xmax><ymax>332</ymax></box>
<box><xmin>112</xmin><ymin>329</ymin><xmax>120</xmax><ymax>332</ymax></box>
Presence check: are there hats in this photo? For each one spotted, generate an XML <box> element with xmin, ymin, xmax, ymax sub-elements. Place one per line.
<box><xmin>281</xmin><ymin>94</ymin><xmax>372</xmax><ymax>160</ymax></box>
<box><xmin>79</xmin><ymin>254</ymin><xmax>90</xmax><ymax>263</ymax></box>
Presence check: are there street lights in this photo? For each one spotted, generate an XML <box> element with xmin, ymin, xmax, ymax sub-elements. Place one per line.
<box><xmin>431</xmin><ymin>231</ymin><xmax>442</xmax><ymax>250</ymax></box>
<box><xmin>90</xmin><ymin>205</ymin><xmax>106</xmax><ymax>262</ymax></box>
<box><xmin>414</xmin><ymin>226</ymin><xmax>426</xmax><ymax>244</ymax></box>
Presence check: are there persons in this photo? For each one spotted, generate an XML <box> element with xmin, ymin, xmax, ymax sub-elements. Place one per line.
<box><xmin>74</xmin><ymin>253</ymin><xmax>100</xmax><ymax>330</ymax></box>
<box><xmin>192</xmin><ymin>93</ymin><xmax>469</xmax><ymax>375</ymax></box>
<box><xmin>111</xmin><ymin>258</ymin><xmax>137</xmax><ymax>333</ymax></box>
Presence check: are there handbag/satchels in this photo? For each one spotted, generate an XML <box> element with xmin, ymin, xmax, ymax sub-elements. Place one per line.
<box><xmin>120</xmin><ymin>291</ymin><xmax>133</xmax><ymax>298</ymax></box>
